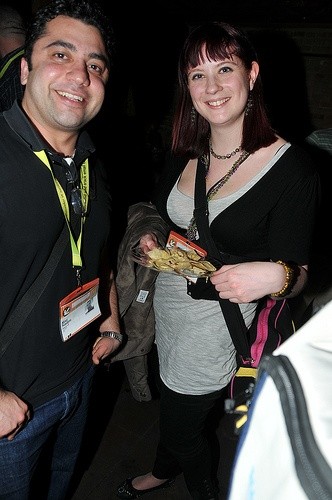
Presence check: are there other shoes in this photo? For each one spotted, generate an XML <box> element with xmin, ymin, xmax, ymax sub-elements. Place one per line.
<box><xmin>114</xmin><ymin>472</ymin><xmax>176</xmax><ymax>499</ymax></box>
<box><xmin>201</xmin><ymin>478</ymin><xmax>219</xmax><ymax>500</ymax></box>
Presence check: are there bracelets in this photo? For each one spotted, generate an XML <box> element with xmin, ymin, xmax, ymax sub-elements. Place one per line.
<box><xmin>101</xmin><ymin>331</ymin><xmax>123</xmax><ymax>342</ymax></box>
<box><xmin>271</xmin><ymin>260</ymin><xmax>294</xmax><ymax>296</ymax></box>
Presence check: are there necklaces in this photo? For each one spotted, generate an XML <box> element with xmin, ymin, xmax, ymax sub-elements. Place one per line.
<box><xmin>185</xmin><ymin>138</ymin><xmax>250</xmax><ymax>241</ymax></box>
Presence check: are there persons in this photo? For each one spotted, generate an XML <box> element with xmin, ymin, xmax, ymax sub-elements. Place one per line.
<box><xmin>0</xmin><ymin>0</ymin><xmax>123</xmax><ymax>500</ymax></box>
<box><xmin>0</xmin><ymin>6</ymin><xmax>26</xmax><ymax>111</ymax></box>
<box><xmin>117</xmin><ymin>20</ymin><xmax>332</xmax><ymax>500</ymax></box>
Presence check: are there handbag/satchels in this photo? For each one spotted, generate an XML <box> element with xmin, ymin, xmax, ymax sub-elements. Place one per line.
<box><xmin>231</xmin><ymin>361</ymin><xmax>259</xmax><ymax>429</ymax></box>
